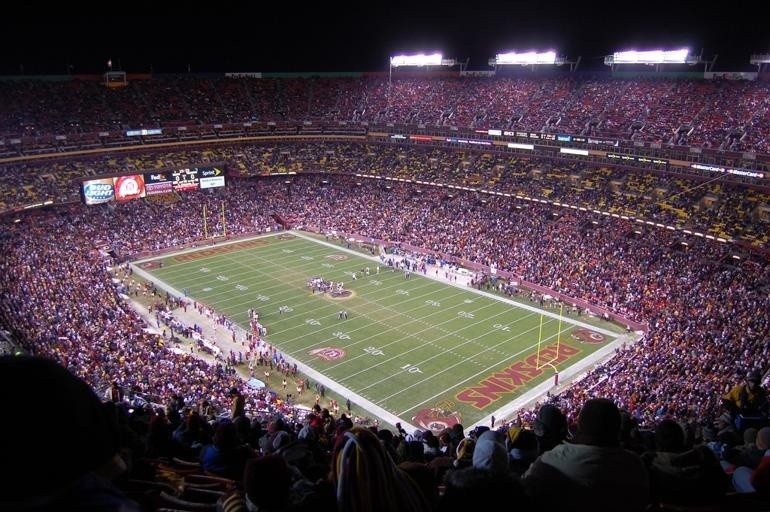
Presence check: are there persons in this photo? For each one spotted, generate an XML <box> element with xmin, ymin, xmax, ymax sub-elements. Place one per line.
<box><xmin>0</xmin><ymin>73</ymin><xmax>770</xmax><ymax>511</ymax></box>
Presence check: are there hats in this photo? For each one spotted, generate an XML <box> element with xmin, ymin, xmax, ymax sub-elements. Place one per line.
<box><xmin>744</xmin><ymin>427</ymin><xmax>769</xmax><ymax>449</ymax></box>
<box><xmin>652</xmin><ymin>419</ymin><xmax>686</xmax><ymax>454</ymax></box>
<box><xmin>702</xmin><ymin>413</ymin><xmax>732</xmax><ymax>440</ymax></box>
<box><xmin>457</xmin><ymin>430</ymin><xmax>509</xmax><ymax>477</ymax></box>
<box><xmin>533</xmin><ymin>404</ymin><xmax>568</xmax><ymax>438</ymax></box>
<box><xmin>576</xmin><ymin>399</ymin><xmax>622</xmax><ymax>439</ymax></box>
